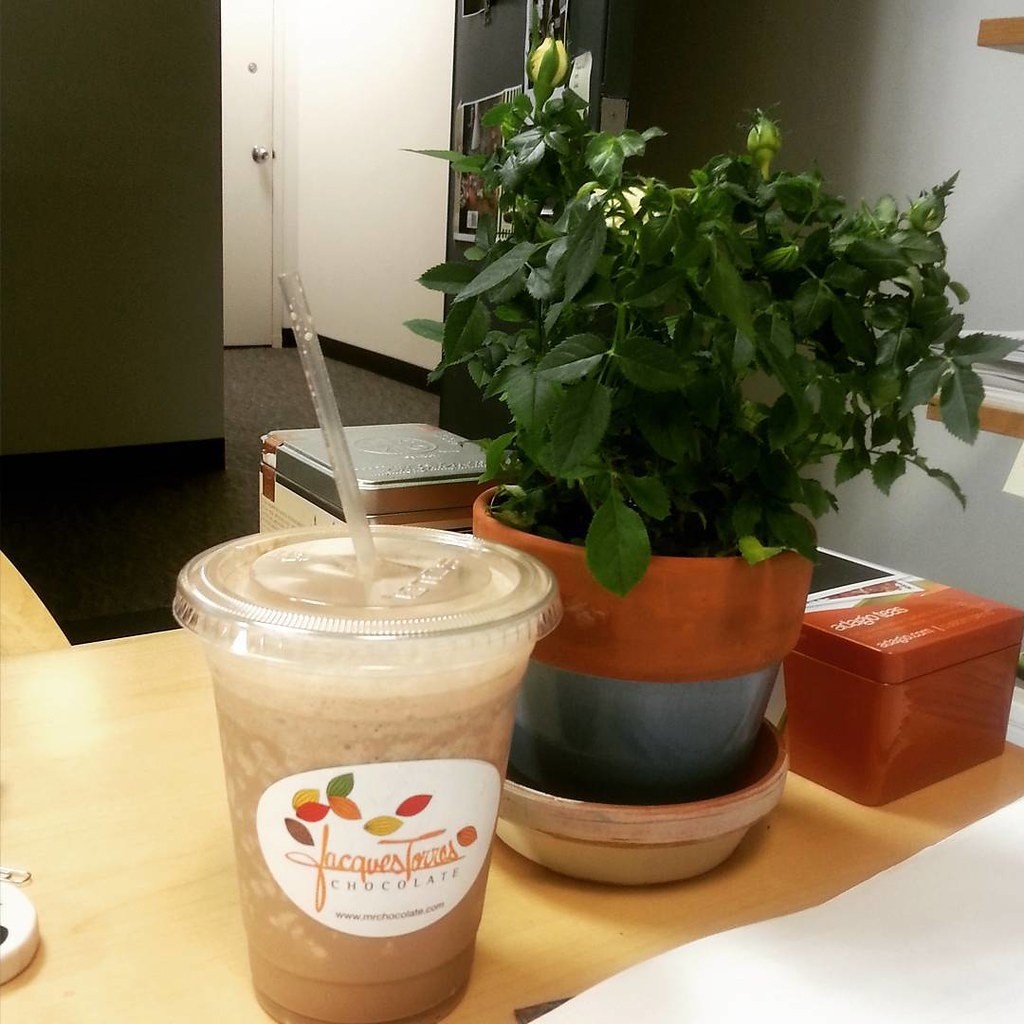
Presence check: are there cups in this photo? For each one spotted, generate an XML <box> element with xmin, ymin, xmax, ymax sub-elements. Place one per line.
<box><xmin>170</xmin><ymin>521</ymin><xmax>565</xmax><ymax>1024</ymax></box>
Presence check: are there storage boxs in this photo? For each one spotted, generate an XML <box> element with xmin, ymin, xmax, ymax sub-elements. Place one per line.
<box><xmin>253</xmin><ymin>425</ymin><xmax>497</xmax><ymax>555</ymax></box>
<box><xmin>782</xmin><ymin>573</ymin><xmax>1024</xmax><ymax>808</ymax></box>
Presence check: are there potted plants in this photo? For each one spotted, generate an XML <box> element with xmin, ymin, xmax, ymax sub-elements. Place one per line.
<box><xmin>406</xmin><ymin>7</ymin><xmax>1024</xmax><ymax>886</ymax></box>
